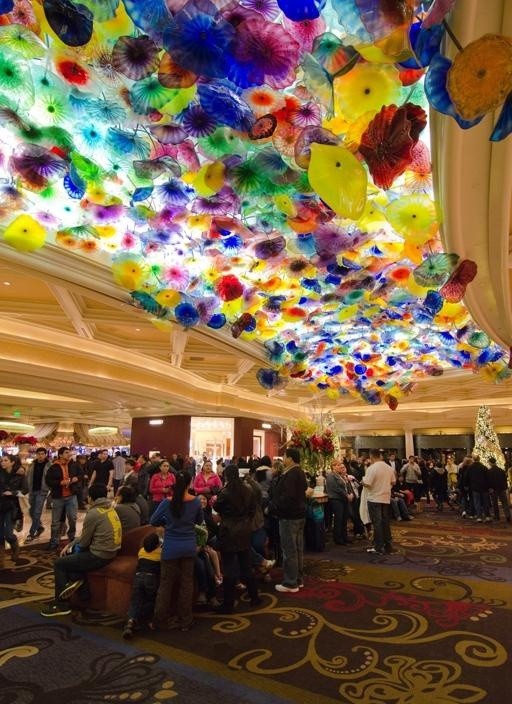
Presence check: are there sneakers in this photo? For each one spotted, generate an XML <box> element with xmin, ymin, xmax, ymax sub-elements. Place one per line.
<box><xmin>366</xmin><ymin>545</ymin><xmax>385</xmax><ymax>555</ymax></box>
<box><xmin>275</xmin><ymin>584</ymin><xmax>300</xmax><ymax>593</ymax></box>
<box><xmin>122</xmin><ymin>616</ymin><xmax>197</xmax><ymax>640</ymax></box>
<box><xmin>355</xmin><ymin>533</ymin><xmax>364</xmax><ymax>540</ymax></box>
<box><xmin>24</xmin><ymin>526</ymin><xmax>76</xmax><ymax>551</ymax></box>
<box><xmin>59</xmin><ymin>578</ymin><xmax>85</xmax><ymax>601</ymax></box>
<box><xmin>459</xmin><ymin>507</ymin><xmax>511</xmax><ymax>523</ymax></box>
<box><xmin>40</xmin><ymin>599</ymin><xmax>72</xmax><ymax>617</ymax></box>
<box><xmin>197</xmin><ymin>558</ymin><xmax>276</xmax><ymax>615</ymax></box>
<box><xmin>394</xmin><ymin>500</ymin><xmax>454</xmax><ymax>524</ymax></box>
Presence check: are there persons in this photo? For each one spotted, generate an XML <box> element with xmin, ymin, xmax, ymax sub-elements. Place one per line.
<box><xmin>41</xmin><ymin>482</ymin><xmax>123</xmax><ymax>618</ymax></box>
<box><xmin>152</xmin><ymin>470</ymin><xmax>207</xmax><ymax>632</ymax></box>
<box><xmin>125</xmin><ymin>533</ymin><xmax>163</xmax><ymax>640</ymax></box>
<box><xmin>211</xmin><ymin>464</ymin><xmax>264</xmax><ymax>615</ymax></box>
<box><xmin>1</xmin><ymin>447</ymin><xmax>308</xmax><ymax>591</ymax></box>
<box><xmin>300</xmin><ymin>448</ymin><xmax>511</xmax><ymax>553</ymax></box>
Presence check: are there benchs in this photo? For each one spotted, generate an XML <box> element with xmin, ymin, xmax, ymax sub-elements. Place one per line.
<box><xmin>83</xmin><ymin>555</ymin><xmax>138</xmax><ymax>619</ymax></box>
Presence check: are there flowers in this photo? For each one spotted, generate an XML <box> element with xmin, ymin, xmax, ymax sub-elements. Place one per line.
<box><xmin>272</xmin><ymin>410</ymin><xmax>339</xmax><ymax>477</ymax></box>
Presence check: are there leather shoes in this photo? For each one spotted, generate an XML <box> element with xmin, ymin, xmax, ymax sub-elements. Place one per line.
<box><xmin>334</xmin><ymin>541</ymin><xmax>347</xmax><ymax>547</ymax></box>
<box><xmin>344</xmin><ymin>539</ymin><xmax>352</xmax><ymax>544</ymax></box>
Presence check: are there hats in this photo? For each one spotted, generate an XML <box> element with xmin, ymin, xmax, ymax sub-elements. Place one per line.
<box><xmin>223</xmin><ymin>464</ymin><xmax>239</xmax><ymax>475</ymax></box>
<box><xmin>409</xmin><ymin>455</ymin><xmax>416</xmax><ymax>459</ymax></box>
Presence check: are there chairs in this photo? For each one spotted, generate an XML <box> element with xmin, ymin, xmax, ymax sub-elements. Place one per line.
<box><xmin>118</xmin><ymin>523</ymin><xmax>156</xmax><ymax>554</ymax></box>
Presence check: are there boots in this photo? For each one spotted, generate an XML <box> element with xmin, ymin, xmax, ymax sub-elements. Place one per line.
<box><xmin>0</xmin><ymin>546</ymin><xmax>6</xmax><ymax>568</ymax></box>
<box><xmin>10</xmin><ymin>537</ymin><xmax>20</xmax><ymax>562</ymax></box>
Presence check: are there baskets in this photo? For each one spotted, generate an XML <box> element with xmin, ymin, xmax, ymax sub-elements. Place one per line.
<box><xmin>311</xmin><ymin>494</ymin><xmax>328</xmax><ymax>503</ymax></box>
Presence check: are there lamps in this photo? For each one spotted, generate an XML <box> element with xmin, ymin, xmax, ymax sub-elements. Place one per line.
<box><xmin>0</xmin><ymin>421</ymin><xmax>35</xmax><ymax>433</ymax></box>
<box><xmin>88</xmin><ymin>426</ymin><xmax>118</xmax><ymax>437</ymax></box>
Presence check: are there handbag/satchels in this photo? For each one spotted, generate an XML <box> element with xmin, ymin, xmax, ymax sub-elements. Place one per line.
<box><xmin>417</xmin><ymin>479</ymin><xmax>424</xmax><ymax>484</ymax></box>
